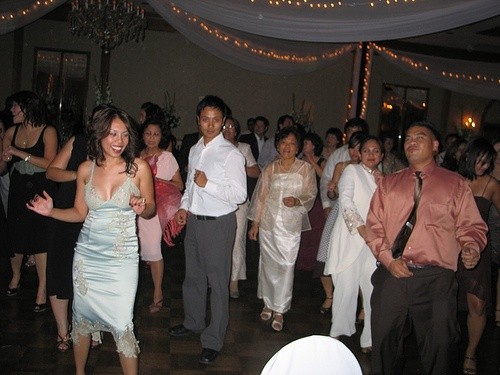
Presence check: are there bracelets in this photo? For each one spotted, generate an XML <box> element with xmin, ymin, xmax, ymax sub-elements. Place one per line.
<box><xmin>24</xmin><ymin>153</ymin><xmax>32</xmax><ymax>162</ymax></box>
<box><xmin>327</xmin><ymin>190</ymin><xmax>336</xmax><ymax>199</ymax></box>
<box><xmin>297</xmin><ymin>199</ymin><xmax>300</xmax><ymax>205</ymax></box>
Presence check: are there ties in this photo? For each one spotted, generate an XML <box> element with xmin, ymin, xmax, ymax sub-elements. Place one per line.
<box><xmin>392</xmin><ymin>172</ymin><xmax>423</xmax><ymax>258</ymax></box>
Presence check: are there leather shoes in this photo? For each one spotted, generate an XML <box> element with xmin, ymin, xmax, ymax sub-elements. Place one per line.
<box><xmin>199</xmin><ymin>348</ymin><xmax>219</xmax><ymax>363</ymax></box>
<box><xmin>169</xmin><ymin>324</ymin><xmax>193</xmax><ymax>336</ymax></box>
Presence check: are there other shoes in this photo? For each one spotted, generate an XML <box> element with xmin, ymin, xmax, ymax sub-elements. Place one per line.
<box><xmin>321</xmin><ymin>297</ymin><xmax>333</xmax><ymax>314</ymax></box>
<box><xmin>150</xmin><ymin>298</ymin><xmax>164</xmax><ymax>313</ymax></box>
<box><xmin>34</xmin><ymin>303</ymin><xmax>47</xmax><ymax>312</ymax></box>
<box><xmin>230</xmin><ymin>287</ymin><xmax>239</xmax><ymax>299</ymax></box>
<box><xmin>7</xmin><ymin>272</ymin><xmax>23</xmax><ymax>295</ymax></box>
<box><xmin>57</xmin><ymin>323</ymin><xmax>72</xmax><ymax>352</ymax></box>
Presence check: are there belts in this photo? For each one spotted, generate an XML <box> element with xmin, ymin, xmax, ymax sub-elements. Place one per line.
<box><xmin>195</xmin><ymin>215</ymin><xmax>216</xmax><ymax>221</ymax></box>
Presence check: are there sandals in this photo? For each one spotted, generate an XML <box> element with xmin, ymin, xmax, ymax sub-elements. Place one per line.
<box><xmin>260</xmin><ymin>306</ymin><xmax>272</xmax><ymax>320</ymax></box>
<box><xmin>463</xmin><ymin>354</ymin><xmax>477</xmax><ymax>374</ymax></box>
<box><xmin>272</xmin><ymin>312</ymin><xmax>284</xmax><ymax>332</ymax></box>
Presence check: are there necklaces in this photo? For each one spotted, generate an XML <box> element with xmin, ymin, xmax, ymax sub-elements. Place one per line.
<box><xmin>280</xmin><ymin>160</ymin><xmax>289</xmax><ymax>172</ymax></box>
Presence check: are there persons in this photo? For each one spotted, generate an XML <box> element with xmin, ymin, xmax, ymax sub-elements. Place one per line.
<box><xmin>0</xmin><ymin>89</ymin><xmax>499</xmax><ymax>374</ymax></box>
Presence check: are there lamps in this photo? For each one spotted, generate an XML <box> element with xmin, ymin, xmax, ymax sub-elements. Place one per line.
<box><xmin>70</xmin><ymin>0</ymin><xmax>148</xmax><ymax>54</ymax></box>
<box><xmin>163</xmin><ymin>89</ymin><xmax>180</xmax><ymax>129</ymax></box>
<box><xmin>92</xmin><ymin>73</ymin><xmax>113</xmax><ymax>104</ymax></box>
<box><xmin>289</xmin><ymin>94</ymin><xmax>314</xmax><ymax>133</ymax></box>
